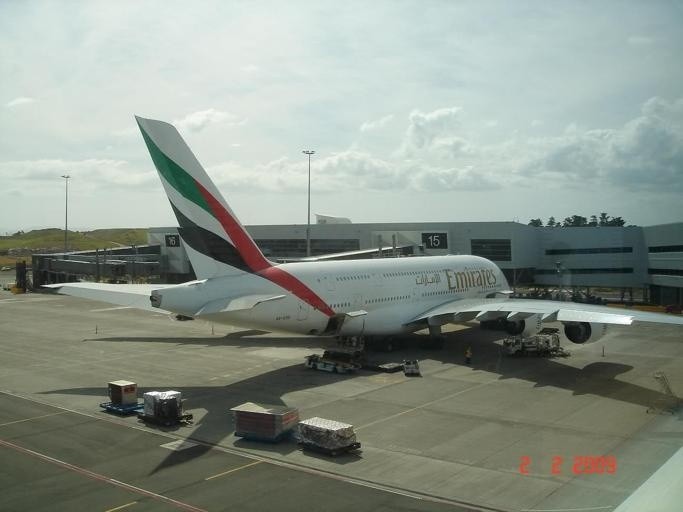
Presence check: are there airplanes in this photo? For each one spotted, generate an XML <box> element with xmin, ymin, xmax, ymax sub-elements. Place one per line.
<box><xmin>39</xmin><ymin>115</ymin><xmax>683</xmax><ymax>367</ymax></box>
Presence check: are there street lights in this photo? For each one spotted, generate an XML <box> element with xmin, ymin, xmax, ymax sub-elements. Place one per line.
<box><xmin>61</xmin><ymin>175</ymin><xmax>72</xmax><ymax>251</ymax></box>
<box><xmin>302</xmin><ymin>150</ymin><xmax>317</xmax><ymax>258</ymax></box>
<box><xmin>556</xmin><ymin>263</ymin><xmax>566</xmax><ymax>301</ymax></box>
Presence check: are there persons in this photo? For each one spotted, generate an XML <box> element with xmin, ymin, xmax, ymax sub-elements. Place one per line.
<box><xmin>465</xmin><ymin>344</ymin><xmax>473</xmax><ymax>364</ymax></box>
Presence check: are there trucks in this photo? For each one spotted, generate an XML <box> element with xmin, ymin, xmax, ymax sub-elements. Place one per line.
<box><xmin>502</xmin><ymin>334</ymin><xmax>560</xmax><ymax>357</ymax></box>
